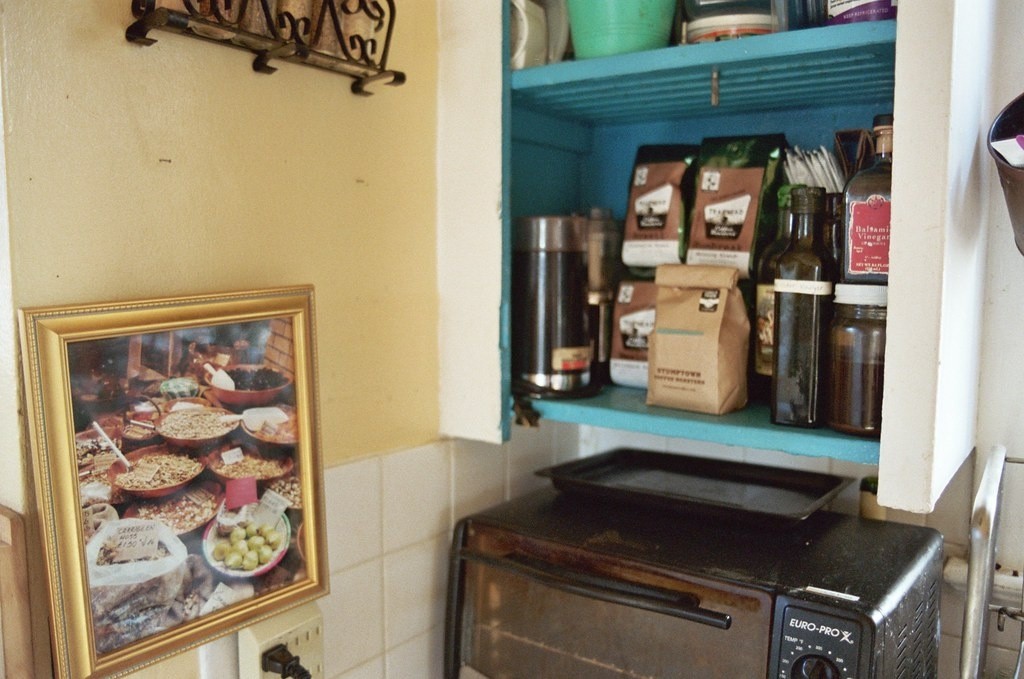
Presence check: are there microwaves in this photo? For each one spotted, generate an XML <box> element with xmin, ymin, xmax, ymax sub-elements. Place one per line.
<box><xmin>443</xmin><ymin>481</ymin><xmax>943</xmax><ymax>679</ymax></box>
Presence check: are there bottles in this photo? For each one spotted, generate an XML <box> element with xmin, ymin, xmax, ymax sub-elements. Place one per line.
<box><xmin>770</xmin><ymin>186</ymin><xmax>833</xmax><ymax>429</ymax></box>
<box><xmin>829</xmin><ymin>284</ymin><xmax>890</xmax><ymax>436</ymax></box>
<box><xmin>510</xmin><ymin>217</ymin><xmax>602</xmax><ymax>400</ymax></box>
<box><xmin>749</xmin><ymin>184</ymin><xmax>809</xmax><ymax>398</ymax></box>
<box><xmin>587</xmin><ymin>208</ymin><xmax>620</xmax><ymax>290</ymax></box>
<box><xmin>841</xmin><ymin>114</ymin><xmax>894</xmax><ymax>280</ymax></box>
<box><xmin>823</xmin><ymin>192</ymin><xmax>843</xmax><ymax>278</ymax></box>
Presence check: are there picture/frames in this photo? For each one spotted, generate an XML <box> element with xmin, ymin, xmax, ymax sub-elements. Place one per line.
<box><xmin>18</xmin><ymin>283</ymin><xmax>328</xmax><ymax>679</ymax></box>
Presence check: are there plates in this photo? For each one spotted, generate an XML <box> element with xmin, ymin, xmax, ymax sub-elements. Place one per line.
<box><xmin>75</xmin><ymin>363</ymin><xmax>307</xmax><ymax>581</ymax></box>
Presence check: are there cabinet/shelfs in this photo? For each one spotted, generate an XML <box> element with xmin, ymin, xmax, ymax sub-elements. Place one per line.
<box><xmin>499</xmin><ymin>0</ymin><xmax>900</xmax><ymax>465</ymax></box>
